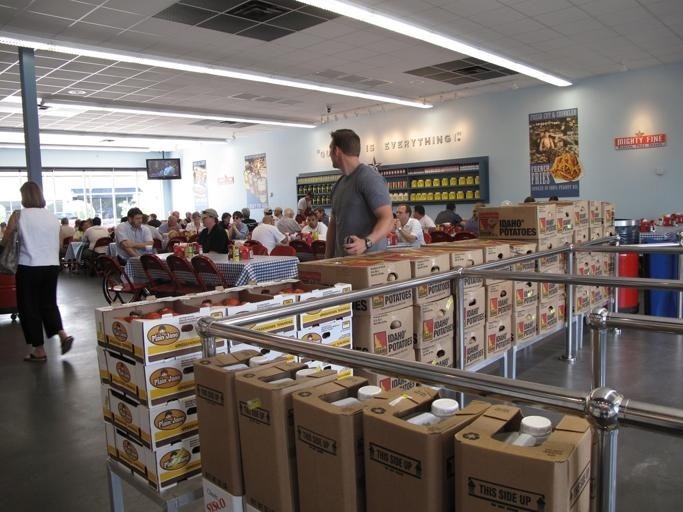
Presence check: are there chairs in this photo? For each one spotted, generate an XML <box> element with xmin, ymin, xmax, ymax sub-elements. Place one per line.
<box><xmin>60</xmin><ymin>237</ymin><xmax>73</xmax><ymax>271</ymax></box>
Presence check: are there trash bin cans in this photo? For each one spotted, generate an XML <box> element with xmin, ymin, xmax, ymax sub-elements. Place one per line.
<box><xmin>642</xmin><ymin>236</ymin><xmax>678</xmax><ymax>318</ymax></box>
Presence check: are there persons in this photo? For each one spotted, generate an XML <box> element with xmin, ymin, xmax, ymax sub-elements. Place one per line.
<box><xmin>322</xmin><ymin>128</ymin><xmax>393</xmax><ymax>258</ymax></box>
<box><xmin>146</xmin><ymin>160</ymin><xmax>179</xmax><ymax>177</ymax></box>
<box><xmin>529</xmin><ymin>116</ymin><xmax>578</xmax><ymax>164</ymax></box>
<box><xmin>0</xmin><ymin>181</ymin><xmax>75</xmax><ymax>365</ymax></box>
<box><xmin>1</xmin><ymin>192</ymin><xmax>560</xmax><ymax>299</ymax></box>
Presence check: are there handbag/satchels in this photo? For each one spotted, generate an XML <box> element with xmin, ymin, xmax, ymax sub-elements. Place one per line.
<box><xmin>0</xmin><ymin>231</ymin><xmax>20</xmax><ymax>275</ymax></box>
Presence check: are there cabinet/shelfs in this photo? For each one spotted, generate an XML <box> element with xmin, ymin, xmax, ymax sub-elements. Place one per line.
<box><xmin>296</xmin><ymin>155</ymin><xmax>489</xmax><ymax>207</ymax></box>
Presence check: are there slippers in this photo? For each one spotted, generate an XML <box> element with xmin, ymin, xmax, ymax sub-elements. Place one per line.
<box><xmin>24</xmin><ymin>353</ymin><xmax>47</xmax><ymax>360</ymax></box>
<box><xmin>62</xmin><ymin>336</ymin><xmax>73</xmax><ymax>354</ymax></box>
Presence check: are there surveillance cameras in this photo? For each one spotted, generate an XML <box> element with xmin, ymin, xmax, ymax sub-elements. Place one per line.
<box><xmin>327</xmin><ymin>105</ymin><xmax>332</xmax><ymax>113</ymax></box>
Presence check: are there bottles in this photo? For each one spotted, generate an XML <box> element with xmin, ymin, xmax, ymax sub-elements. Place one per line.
<box><xmin>227</xmin><ymin>242</ymin><xmax>253</xmax><ymax>262</ymax></box>
<box><xmin>299</xmin><ymin>183</ymin><xmax>335</xmax><ymax>193</ymax></box>
<box><xmin>288</xmin><ymin>227</ymin><xmax>319</xmax><ymax>244</ymax></box>
<box><xmin>174</xmin><ymin>241</ymin><xmax>204</xmax><ymax>258</ymax></box>
<box><xmin>384</xmin><ymin>178</ymin><xmax>407</xmax><ymax>188</ymax></box>
<box><xmin>311</xmin><ymin>195</ymin><xmax>332</xmax><ymax>204</ymax></box>
<box><xmin>433</xmin><ymin>223</ymin><xmax>465</xmax><ymax>234</ymax></box>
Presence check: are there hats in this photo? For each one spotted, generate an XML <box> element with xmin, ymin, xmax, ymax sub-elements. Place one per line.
<box><xmin>202</xmin><ymin>208</ymin><xmax>218</xmax><ymax>218</ymax></box>
<box><xmin>242</xmin><ymin>208</ymin><xmax>250</xmax><ymax>216</ymax></box>
<box><xmin>264</xmin><ymin>208</ymin><xmax>272</xmax><ymax>213</ymax></box>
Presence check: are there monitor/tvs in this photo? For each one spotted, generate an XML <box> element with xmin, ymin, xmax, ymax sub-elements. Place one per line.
<box><xmin>145</xmin><ymin>159</ymin><xmax>182</xmax><ymax>180</ymax></box>
